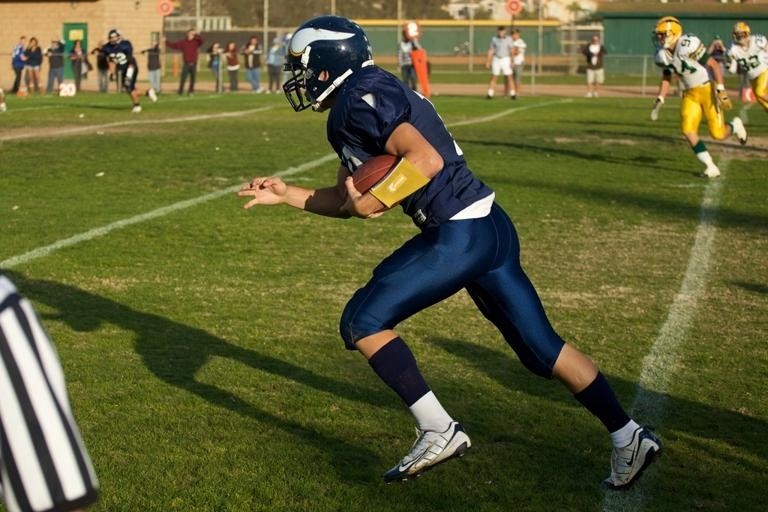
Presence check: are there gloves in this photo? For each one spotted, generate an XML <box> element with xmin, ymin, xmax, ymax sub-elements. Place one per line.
<box><xmin>716</xmin><ymin>83</ymin><xmax>733</xmax><ymax>110</ymax></box>
<box><xmin>650</xmin><ymin>96</ymin><xmax>665</xmax><ymax>119</ymax></box>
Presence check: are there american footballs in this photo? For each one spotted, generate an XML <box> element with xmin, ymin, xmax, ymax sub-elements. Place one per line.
<box><xmin>340</xmin><ymin>155</ymin><xmax>399</xmax><ymax>213</ymax></box>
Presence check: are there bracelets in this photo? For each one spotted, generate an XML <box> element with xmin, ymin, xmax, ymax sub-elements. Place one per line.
<box><xmin>654</xmin><ymin>95</ymin><xmax>665</xmax><ymax>105</ymax></box>
<box><xmin>716</xmin><ymin>85</ymin><xmax>724</xmax><ymax>94</ymax></box>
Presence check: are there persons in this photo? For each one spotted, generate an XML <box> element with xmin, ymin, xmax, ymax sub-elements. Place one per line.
<box><xmin>0</xmin><ymin>273</ymin><xmax>103</xmax><ymax>511</ymax></box>
<box><xmin>708</xmin><ymin>33</ymin><xmax>728</xmax><ymax>79</ymax></box>
<box><xmin>725</xmin><ymin>21</ymin><xmax>768</xmax><ymax>113</ymax></box>
<box><xmin>649</xmin><ymin>15</ymin><xmax>747</xmax><ymax>179</ymax></box>
<box><xmin>395</xmin><ymin>28</ymin><xmax>423</xmax><ymax>91</ymax></box>
<box><xmin>455</xmin><ymin>26</ymin><xmax>527</xmax><ymax>101</ymax></box>
<box><xmin>161</xmin><ymin>28</ymin><xmax>306</xmax><ymax>99</ymax></box>
<box><xmin>236</xmin><ymin>16</ymin><xmax>662</xmax><ymax>487</ymax></box>
<box><xmin>582</xmin><ymin>36</ymin><xmax>608</xmax><ymax>98</ymax></box>
<box><xmin>1</xmin><ymin>28</ymin><xmax>161</xmax><ymax>114</ymax></box>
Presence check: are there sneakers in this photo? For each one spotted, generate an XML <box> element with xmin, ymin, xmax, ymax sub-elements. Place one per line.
<box><xmin>148</xmin><ymin>88</ymin><xmax>157</xmax><ymax>102</ymax></box>
<box><xmin>605</xmin><ymin>425</ymin><xmax>661</xmax><ymax>490</ymax></box>
<box><xmin>382</xmin><ymin>420</ymin><xmax>472</xmax><ymax>482</ymax></box>
<box><xmin>732</xmin><ymin>116</ymin><xmax>747</xmax><ymax>144</ymax></box>
<box><xmin>132</xmin><ymin>105</ymin><xmax>142</xmax><ymax>112</ymax></box>
<box><xmin>698</xmin><ymin>169</ymin><xmax>721</xmax><ymax>177</ymax></box>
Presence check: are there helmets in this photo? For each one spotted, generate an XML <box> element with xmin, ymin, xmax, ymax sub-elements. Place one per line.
<box><xmin>282</xmin><ymin>16</ymin><xmax>374</xmax><ymax>112</ymax></box>
<box><xmin>652</xmin><ymin>16</ymin><xmax>682</xmax><ymax>49</ymax></box>
<box><xmin>109</xmin><ymin>27</ymin><xmax>120</xmax><ymax>43</ymax></box>
<box><xmin>733</xmin><ymin>21</ymin><xmax>751</xmax><ymax>46</ymax></box>
